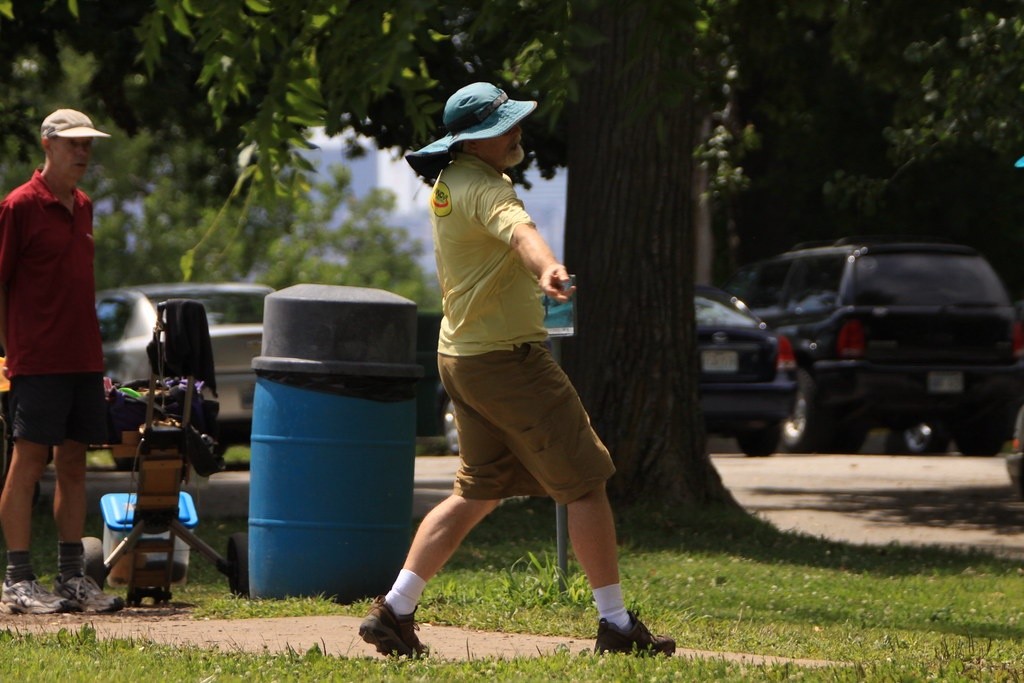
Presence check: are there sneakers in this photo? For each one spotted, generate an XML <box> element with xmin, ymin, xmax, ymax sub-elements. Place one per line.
<box><xmin>53</xmin><ymin>574</ymin><xmax>124</xmax><ymax>611</ymax></box>
<box><xmin>594</xmin><ymin>610</ymin><xmax>676</xmax><ymax>658</ymax></box>
<box><xmin>358</xmin><ymin>595</ymin><xmax>430</xmax><ymax>661</ymax></box>
<box><xmin>0</xmin><ymin>579</ymin><xmax>70</xmax><ymax>616</ymax></box>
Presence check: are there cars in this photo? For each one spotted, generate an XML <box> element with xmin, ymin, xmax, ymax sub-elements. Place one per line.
<box><xmin>414</xmin><ymin>270</ymin><xmax>801</xmax><ymax>458</ymax></box>
<box><xmin>93</xmin><ymin>280</ymin><xmax>275</xmax><ymax>461</ymax></box>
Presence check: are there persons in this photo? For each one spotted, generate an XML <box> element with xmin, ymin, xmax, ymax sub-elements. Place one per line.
<box><xmin>359</xmin><ymin>83</ymin><xmax>678</xmax><ymax>663</ymax></box>
<box><xmin>0</xmin><ymin>108</ymin><xmax>124</xmax><ymax>614</ymax></box>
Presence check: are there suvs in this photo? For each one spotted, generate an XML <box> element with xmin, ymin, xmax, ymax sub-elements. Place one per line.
<box><xmin>727</xmin><ymin>240</ymin><xmax>1024</xmax><ymax>452</ymax></box>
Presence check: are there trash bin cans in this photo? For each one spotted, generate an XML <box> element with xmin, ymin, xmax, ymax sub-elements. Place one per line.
<box><xmin>247</xmin><ymin>284</ymin><xmax>423</xmax><ymax>605</ymax></box>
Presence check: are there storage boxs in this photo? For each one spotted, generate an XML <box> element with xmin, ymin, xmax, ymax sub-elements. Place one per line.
<box><xmin>101</xmin><ymin>491</ymin><xmax>198</xmax><ymax>586</ymax></box>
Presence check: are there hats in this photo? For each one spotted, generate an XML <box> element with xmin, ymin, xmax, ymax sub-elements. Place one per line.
<box><xmin>41</xmin><ymin>108</ymin><xmax>111</xmax><ymax>138</ymax></box>
<box><xmin>405</xmin><ymin>81</ymin><xmax>538</xmax><ymax>181</ymax></box>
